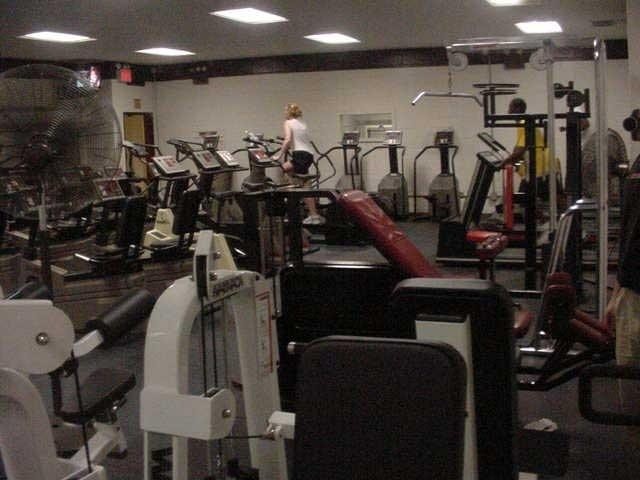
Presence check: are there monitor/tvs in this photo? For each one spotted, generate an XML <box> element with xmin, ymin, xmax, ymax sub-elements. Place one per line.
<box><xmin>57</xmin><ymin>62</ymin><xmax>102</xmax><ymax>99</ymax></box>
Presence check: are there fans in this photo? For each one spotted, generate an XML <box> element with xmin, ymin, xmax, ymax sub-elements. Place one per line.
<box><xmin>1</xmin><ymin>62</ymin><xmax>128</xmax><ymax>417</ymax></box>
<box><xmin>582</xmin><ymin>129</ymin><xmax>631</xmax><ymax>220</ymax></box>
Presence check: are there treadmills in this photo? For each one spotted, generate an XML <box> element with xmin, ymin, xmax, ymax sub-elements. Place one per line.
<box><xmin>148</xmin><ymin>148</ymin><xmax>338</xmax><ymax>257</ymax></box>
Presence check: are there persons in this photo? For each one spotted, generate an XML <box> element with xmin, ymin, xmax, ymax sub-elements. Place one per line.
<box><xmin>272</xmin><ymin>103</ymin><xmax>323</xmax><ymax>225</ymax></box>
<box><xmin>496</xmin><ymin>97</ymin><xmax>550</xmax><ymax>190</ymax></box>
<box><xmin>612</xmin><ymin>107</ymin><xmax>639</xmax><ymax>420</ymax></box>
